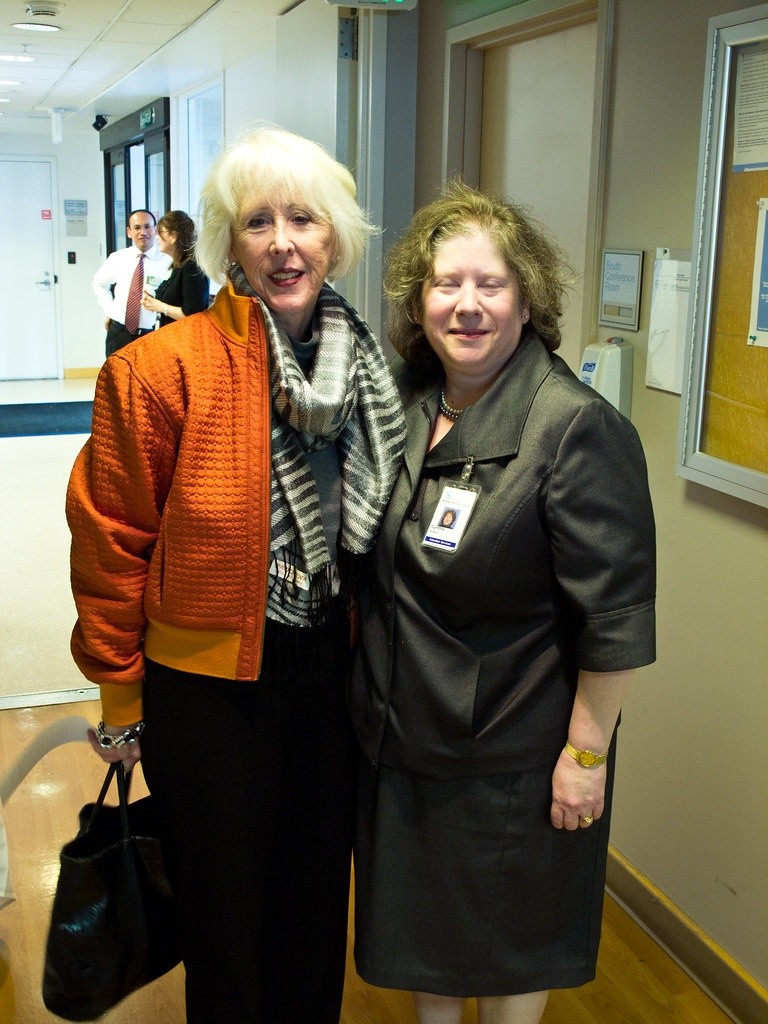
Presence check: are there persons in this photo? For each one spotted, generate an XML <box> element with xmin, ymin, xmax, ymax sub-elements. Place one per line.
<box><xmin>438</xmin><ymin>509</ymin><xmax>456</xmax><ymax>529</ymax></box>
<box><xmin>348</xmin><ymin>189</ymin><xmax>657</xmax><ymax>1024</ymax></box>
<box><xmin>93</xmin><ymin>209</ymin><xmax>174</xmax><ymax>359</ymax></box>
<box><xmin>141</xmin><ymin>211</ymin><xmax>210</xmax><ymax>329</ymax></box>
<box><xmin>65</xmin><ymin>130</ymin><xmax>382</xmax><ymax>1024</ymax></box>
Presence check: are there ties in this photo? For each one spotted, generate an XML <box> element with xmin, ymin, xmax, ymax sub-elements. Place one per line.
<box><xmin>125</xmin><ymin>254</ymin><xmax>147</xmax><ymax>334</ymax></box>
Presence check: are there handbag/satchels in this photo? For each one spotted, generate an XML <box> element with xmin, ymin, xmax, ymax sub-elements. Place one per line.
<box><xmin>41</xmin><ymin>759</ymin><xmax>186</xmax><ymax>1023</ymax></box>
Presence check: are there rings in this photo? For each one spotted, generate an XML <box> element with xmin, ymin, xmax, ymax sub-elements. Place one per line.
<box><xmin>580</xmin><ymin>816</ymin><xmax>593</xmax><ymax>825</ymax></box>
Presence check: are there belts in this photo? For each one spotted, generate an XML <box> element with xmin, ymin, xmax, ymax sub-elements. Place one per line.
<box><xmin>110</xmin><ymin>319</ymin><xmax>153</xmax><ymax>335</ymax></box>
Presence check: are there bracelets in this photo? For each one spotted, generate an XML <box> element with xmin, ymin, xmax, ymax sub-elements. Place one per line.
<box><xmin>98</xmin><ymin>721</ymin><xmax>144</xmax><ymax>750</ymax></box>
<box><xmin>164</xmin><ymin>304</ymin><xmax>168</xmax><ymax>316</ymax></box>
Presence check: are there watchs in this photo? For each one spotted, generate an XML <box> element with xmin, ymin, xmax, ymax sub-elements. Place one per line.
<box><xmin>565</xmin><ymin>742</ymin><xmax>608</xmax><ymax>768</ymax></box>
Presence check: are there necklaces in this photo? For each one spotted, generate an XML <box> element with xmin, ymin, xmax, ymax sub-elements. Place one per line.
<box><xmin>438</xmin><ymin>389</ymin><xmax>463</xmax><ymax>420</ymax></box>
